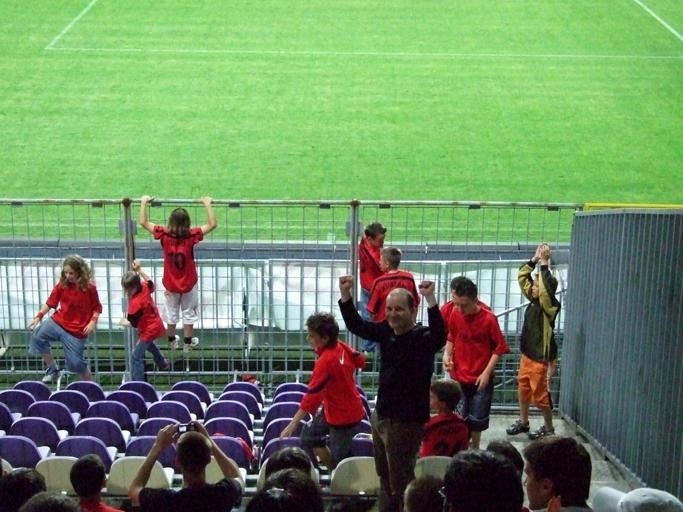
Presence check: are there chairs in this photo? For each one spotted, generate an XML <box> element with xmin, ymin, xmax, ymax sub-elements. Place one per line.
<box><xmin>1</xmin><ymin>375</ymin><xmax>483</xmax><ymax>511</ymax></box>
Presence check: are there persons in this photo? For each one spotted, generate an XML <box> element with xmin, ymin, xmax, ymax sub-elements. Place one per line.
<box><xmin>355</xmin><ymin>221</ymin><xmax>385</xmax><ymax>354</ymax></box>
<box><xmin>437</xmin><ymin>276</ymin><xmax>499</xmax><ymax>338</ymax></box>
<box><xmin>419</xmin><ymin>378</ymin><xmax>470</xmax><ymax>457</ymax></box>
<box><xmin>506</xmin><ymin>240</ymin><xmax>561</xmax><ymax>441</ymax></box>
<box><xmin>336</xmin><ymin>274</ymin><xmax>448</xmax><ymax>510</ymax></box>
<box><xmin>138</xmin><ymin>195</ymin><xmax>218</xmax><ymax>352</ymax></box>
<box><xmin>246</xmin><ymin>450</ymin><xmax>325</xmax><ymax>512</ymax></box>
<box><xmin>440</xmin><ymin>283</ymin><xmax>510</xmax><ymax>451</ymax></box>
<box><xmin>1</xmin><ymin>421</ymin><xmax>245</xmax><ymax>512</ymax></box>
<box><xmin>279</xmin><ymin>311</ymin><xmax>366</xmax><ymax>473</ymax></box>
<box><xmin>23</xmin><ymin>252</ymin><xmax>101</xmax><ymax>383</ymax></box>
<box><xmin>364</xmin><ymin>247</ymin><xmax>421</xmax><ymax>366</ymax></box>
<box><xmin>372</xmin><ymin>437</ymin><xmax>592</xmax><ymax>512</ymax></box>
<box><xmin>117</xmin><ymin>258</ymin><xmax>168</xmax><ymax>380</ymax></box>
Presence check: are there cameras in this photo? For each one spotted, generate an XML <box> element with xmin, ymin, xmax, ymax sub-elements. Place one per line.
<box><xmin>177</xmin><ymin>423</ymin><xmax>195</xmax><ymax>433</ymax></box>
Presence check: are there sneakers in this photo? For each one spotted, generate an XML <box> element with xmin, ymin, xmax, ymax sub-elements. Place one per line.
<box><xmin>42</xmin><ymin>368</ymin><xmax>58</xmax><ymax>383</ymax></box>
<box><xmin>506</xmin><ymin>419</ymin><xmax>529</xmax><ymax>435</ymax></box>
<box><xmin>183</xmin><ymin>337</ymin><xmax>198</xmax><ymax>353</ymax></box>
<box><xmin>529</xmin><ymin>426</ymin><xmax>554</xmax><ymax>439</ymax></box>
<box><xmin>169</xmin><ymin>335</ymin><xmax>179</xmax><ymax>349</ymax></box>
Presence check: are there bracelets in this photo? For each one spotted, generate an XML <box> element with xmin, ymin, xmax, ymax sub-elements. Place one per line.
<box><xmin>90</xmin><ymin>315</ymin><xmax>99</xmax><ymax>323</ymax></box>
<box><xmin>34</xmin><ymin>311</ymin><xmax>43</xmax><ymax>320</ymax></box>
